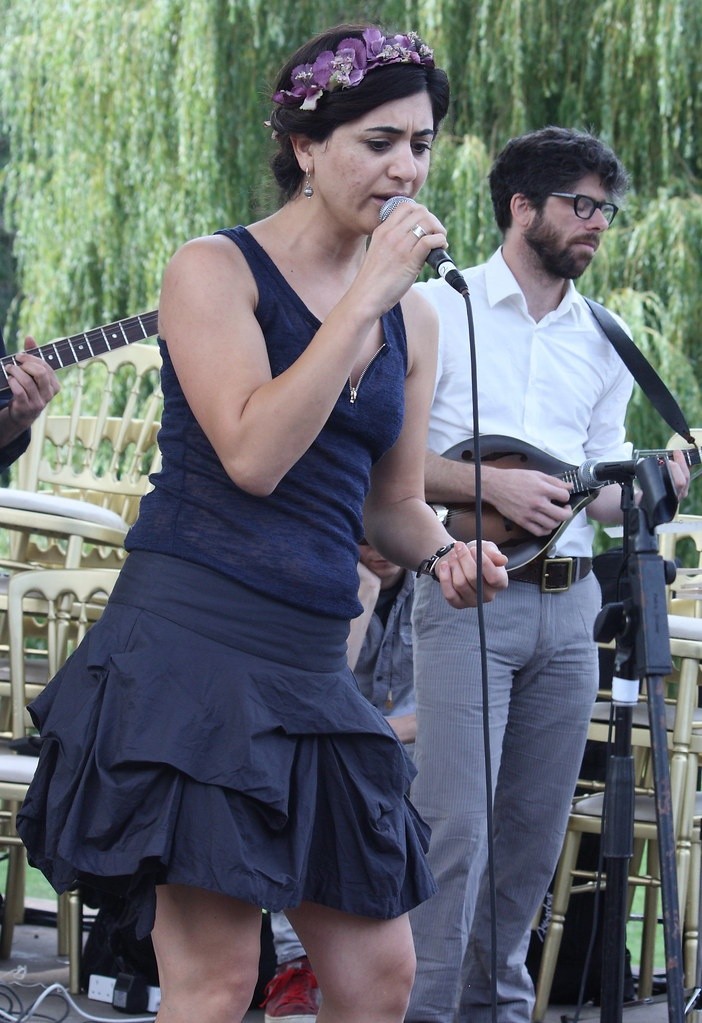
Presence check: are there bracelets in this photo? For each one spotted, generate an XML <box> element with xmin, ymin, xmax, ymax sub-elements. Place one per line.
<box><xmin>416</xmin><ymin>542</ymin><xmax>454</xmax><ymax>583</ymax></box>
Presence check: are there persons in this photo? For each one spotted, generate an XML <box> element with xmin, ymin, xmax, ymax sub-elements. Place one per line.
<box><xmin>17</xmin><ymin>24</ymin><xmax>510</xmax><ymax>1023</ymax></box>
<box><xmin>257</xmin><ymin>528</ymin><xmax>419</xmax><ymax>1023</ymax></box>
<box><xmin>0</xmin><ymin>331</ymin><xmax>60</xmax><ymax>474</ymax></box>
<box><xmin>410</xmin><ymin>127</ymin><xmax>689</xmax><ymax>1023</ymax></box>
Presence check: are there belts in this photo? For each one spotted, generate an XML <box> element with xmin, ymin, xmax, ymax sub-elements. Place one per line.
<box><xmin>506</xmin><ymin>555</ymin><xmax>593</xmax><ymax>593</ymax></box>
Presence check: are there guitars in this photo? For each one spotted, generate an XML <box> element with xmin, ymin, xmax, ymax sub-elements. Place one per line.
<box><xmin>426</xmin><ymin>434</ymin><xmax>702</xmax><ymax>572</ymax></box>
<box><xmin>0</xmin><ymin>309</ymin><xmax>159</xmax><ymax>392</ymax></box>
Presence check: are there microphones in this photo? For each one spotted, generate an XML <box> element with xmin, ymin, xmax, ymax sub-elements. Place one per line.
<box><xmin>378</xmin><ymin>195</ymin><xmax>463</xmax><ymax>292</ymax></box>
<box><xmin>578</xmin><ymin>459</ymin><xmax>642</xmax><ymax>489</ymax></box>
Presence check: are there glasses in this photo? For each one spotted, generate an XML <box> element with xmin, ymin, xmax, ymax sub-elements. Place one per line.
<box><xmin>549</xmin><ymin>189</ymin><xmax>619</xmax><ymax>227</ymax></box>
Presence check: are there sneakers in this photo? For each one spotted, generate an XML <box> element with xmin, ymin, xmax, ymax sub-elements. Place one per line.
<box><xmin>259</xmin><ymin>958</ymin><xmax>319</xmax><ymax>1023</ymax></box>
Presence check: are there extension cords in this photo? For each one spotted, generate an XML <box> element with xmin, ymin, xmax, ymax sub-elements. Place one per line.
<box><xmin>87</xmin><ymin>974</ymin><xmax>162</xmax><ymax>1013</ymax></box>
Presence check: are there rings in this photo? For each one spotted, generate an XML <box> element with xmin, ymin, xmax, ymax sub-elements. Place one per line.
<box><xmin>411</xmin><ymin>223</ymin><xmax>426</xmax><ymax>239</ymax></box>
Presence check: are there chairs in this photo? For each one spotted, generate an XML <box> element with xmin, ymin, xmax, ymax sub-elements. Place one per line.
<box><xmin>533</xmin><ymin>514</ymin><xmax>702</xmax><ymax>1023</ymax></box>
<box><xmin>0</xmin><ymin>339</ymin><xmax>163</xmax><ymax>732</ymax></box>
<box><xmin>0</xmin><ymin>569</ymin><xmax>137</xmax><ymax>993</ymax></box>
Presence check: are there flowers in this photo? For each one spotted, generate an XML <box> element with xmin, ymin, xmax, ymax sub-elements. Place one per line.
<box><xmin>263</xmin><ymin>26</ymin><xmax>434</xmax><ymax>139</ymax></box>
